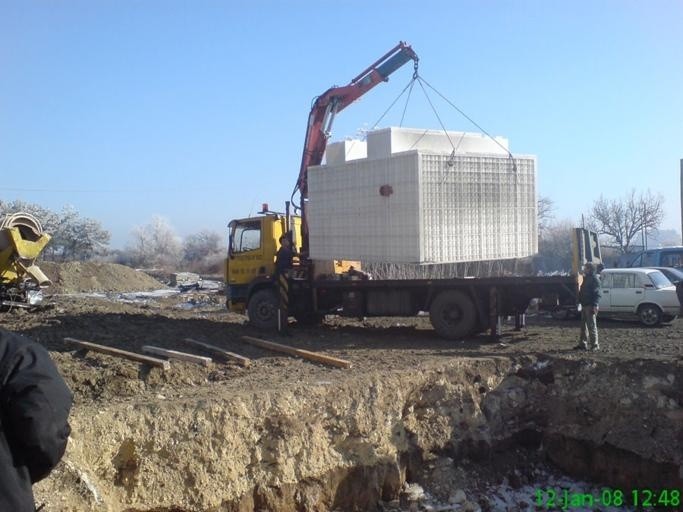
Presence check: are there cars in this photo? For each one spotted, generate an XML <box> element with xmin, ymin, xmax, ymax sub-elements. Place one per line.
<box><xmin>550</xmin><ymin>246</ymin><xmax>682</xmax><ymax>327</ymax></box>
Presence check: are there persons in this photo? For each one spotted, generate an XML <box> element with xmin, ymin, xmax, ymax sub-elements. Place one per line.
<box><xmin>272</xmin><ymin>235</ymin><xmax>300</xmax><ymax>289</ymax></box>
<box><xmin>0</xmin><ymin>320</ymin><xmax>74</xmax><ymax>512</ymax></box>
<box><xmin>571</xmin><ymin>260</ymin><xmax>604</xmax><ymax>353</ymax></box>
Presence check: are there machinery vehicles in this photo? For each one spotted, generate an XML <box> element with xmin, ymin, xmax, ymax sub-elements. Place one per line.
<box><xmin>220</xmin><ymin>41</ymin><xmax>602</xmax><ymax>341</ymax></box>
<box><xmin>0</xmin><ymin>212</ymin><xmax>51</xmax><ymax>312</ymax></box>
